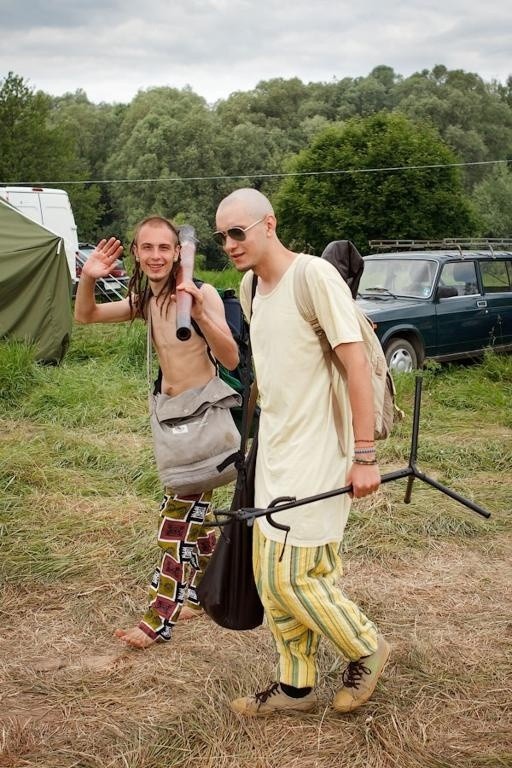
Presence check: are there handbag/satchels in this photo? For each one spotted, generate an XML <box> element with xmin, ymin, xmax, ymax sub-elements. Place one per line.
<box><xmin>148</xmin><ymin>377</ymin><xmax>246</xmax><ymax>495</ymax></box>
<box><xmin>195</xmin><ymin>452</ymin><xmax>264</xmax><ymax>630</ymax></box>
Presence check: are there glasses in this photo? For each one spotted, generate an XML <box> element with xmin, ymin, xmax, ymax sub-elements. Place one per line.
<box><xmin>212</xmin><ymin>218</ymin><xmax>264</xmax><ymax>246</ymax></box>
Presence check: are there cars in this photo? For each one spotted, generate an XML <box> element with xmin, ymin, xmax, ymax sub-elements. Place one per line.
<box><xmin>72</xmin><ymin>243</ymin><xmax>130</xmax><ymax>298</ymax></box>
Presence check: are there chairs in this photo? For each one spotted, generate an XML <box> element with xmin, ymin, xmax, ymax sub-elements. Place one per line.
<box><xmin>451</xmin><ymin>262</ymin><xmax>477</xmax><ymax>295</ymax></box>
<box><xmin>410</xmin><ymin>261</ymin><xmax>436</xmax><ymax>283</ymax></box>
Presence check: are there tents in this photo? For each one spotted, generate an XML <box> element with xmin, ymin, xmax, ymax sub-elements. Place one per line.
<box><xmin>1</xmin><ymin>197</ymin><xmax>79</xmax><ymax>367</ymax></box>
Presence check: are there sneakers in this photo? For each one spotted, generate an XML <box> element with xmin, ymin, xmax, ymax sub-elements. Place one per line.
<box><xmin>332</xmin><ymin>636</ymin><xmax>390</xmax><ymax>713</ymax></box>
<box><xmin>232</xmin><ymin>681</ymin><xmax>318</xmax><ymax>715</ymax></box>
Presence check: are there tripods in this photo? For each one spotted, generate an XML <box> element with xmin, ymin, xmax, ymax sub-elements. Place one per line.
<box><xmin>200</xmin><ymin>375</ymin><xmax>491</xmax><ymax>544</ymax></box>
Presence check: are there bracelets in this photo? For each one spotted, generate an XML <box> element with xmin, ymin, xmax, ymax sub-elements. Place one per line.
<box><xmin>354</xmin><ymin>439</ymin><xmax>378</xmax><ymax>443</ymax></box>
<box><xmin>352</xmin><ymin>445</ymin><xmax>377</xmax><ymax>453</ymax></box>
<box><xmin>350</xmin><ymin>457</ymin><xmax>378</xmax><ymax>466</ymax></box>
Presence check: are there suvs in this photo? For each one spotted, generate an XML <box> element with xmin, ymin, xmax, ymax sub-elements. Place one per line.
<box><xmin>352</xmin><ymin>240</ymin><xmax>512</xmax><ymax>376</ymax></box>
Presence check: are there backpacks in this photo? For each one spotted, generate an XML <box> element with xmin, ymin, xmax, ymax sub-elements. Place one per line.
<box><xmin>293</xmin><ymin>254</ymin><xmax>403</xmax><ymax>456</ymax></box>
<box><xmin>191</xmin><ymin>278</ymin><xmax>261</xmax><ymax>439</ymax></box>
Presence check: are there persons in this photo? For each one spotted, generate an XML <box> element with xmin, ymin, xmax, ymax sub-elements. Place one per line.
<box><xmin>211</xmin><ymin>185</ymin><xmax>391</xmax><ymax>719</ymax></box>
<box><xmin>72</xmin><ymin>215</ymin><xmax>244</xmax><ymax>647</ymax></box>
